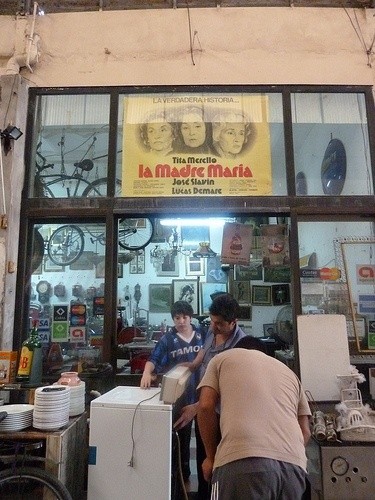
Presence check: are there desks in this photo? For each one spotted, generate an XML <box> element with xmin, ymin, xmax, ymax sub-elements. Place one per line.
<box><xmin>275</xmin><ymin>349</ymin><xmax>295</xmax><ymax>371</ymax></box>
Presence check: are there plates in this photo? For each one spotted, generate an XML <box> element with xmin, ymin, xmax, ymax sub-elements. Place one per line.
<box><xmin>54</xmin><ymin>381</ymin><xmax>87</xmax><ymax>417</ymax></box>
<box><xmin>0</xmin><ymin>404</ymin><xmax>34</xmax><ymax>433</ymax></box>
<box><xmin>32</xmin><ymin>385</ymin><xmax>71</xmax><ymax>432</ymax></box>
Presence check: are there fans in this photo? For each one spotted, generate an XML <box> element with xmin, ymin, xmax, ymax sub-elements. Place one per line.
<box><xmin>276</xmin><ymin>305</ymin><xmax>293</xmax><ymax>342</ymax></box>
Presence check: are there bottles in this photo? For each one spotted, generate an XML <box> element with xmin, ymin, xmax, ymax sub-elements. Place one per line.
<box><xmin>16</xmin><ymin>326</ymin><xmax>44</xmax><ymax>382</ymax></box>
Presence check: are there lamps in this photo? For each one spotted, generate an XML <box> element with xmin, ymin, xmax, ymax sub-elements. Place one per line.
<box><xmin>193</xmin><ymin>225</ymin><xmax>217</xmax><ymax>258</ymax></box>
<box><xmin>1</xmin><ymin>126</ymin><xmax>23</xmax><ymax>140</ymax></box>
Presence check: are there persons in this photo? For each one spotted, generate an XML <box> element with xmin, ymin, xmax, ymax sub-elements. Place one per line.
<box><xmin>173</xmin><ymin>293</ymin><xmax>248</xmax><ymax>500</ymax></box>
<box><xmin>179</xmin><ymin>285</ymin><xmax>194</xmax><ymax>304</ymax></box>
<box><xmin>195</xmin><ymin>335</ymin><xmax>313</xmax><ymax>500</ymax></box>
<box><xmin>139</xmin><ymin>107</ymin><xmax>252</xmax><ymax>159</ymax></box>
<box><xmin>140</xmin><ymin>301</ymin><xmax>206</xmax><ymax>500</ymax></box>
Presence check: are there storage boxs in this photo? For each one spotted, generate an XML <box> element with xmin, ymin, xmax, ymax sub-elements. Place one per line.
<box><xmin>299</xmin><ymin>269</ymin><xmax>321</xmax><ymax>278</ymax></box>
<box><xmin>0</xmin><ymin>351</ymin><xmax>17</xmax><ymax>383</ymax></box>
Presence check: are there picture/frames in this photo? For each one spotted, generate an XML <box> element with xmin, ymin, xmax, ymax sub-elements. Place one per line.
<box><xmin>264</xmin><ymin>323</ymin><xmax>278</xmax><ymax>336</ymax></box>
<box><xmin>30</xmin><ymin>218</ymin><xmax>290</xmax><ymax>322</ymax></box>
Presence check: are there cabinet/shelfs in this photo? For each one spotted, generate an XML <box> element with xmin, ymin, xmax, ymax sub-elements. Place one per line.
<box><xmin>0</xmin><ymin>413</ymin><xmax>88</xmax><ymax>500</ymax></box>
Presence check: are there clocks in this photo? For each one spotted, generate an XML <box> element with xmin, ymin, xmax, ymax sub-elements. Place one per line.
<box><xmin>37</xmin><ymin>281</ymin><xmax>50</xmax><ymax>304</ymax></box>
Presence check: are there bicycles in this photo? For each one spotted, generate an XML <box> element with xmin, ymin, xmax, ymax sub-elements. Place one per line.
<box><xmin>0</xmin><ymin>411</ymin><xmax>71</xmax><ymax>500</ymax></box>
<box><xmin>47</xmin><ymin>216</ymin><xmax>155</xmax><ymax>266</ymax></box>
<box><xmin>34</xmin><ymin>141</ymin><xmax>128</xmax><ymax>225</ymax></box>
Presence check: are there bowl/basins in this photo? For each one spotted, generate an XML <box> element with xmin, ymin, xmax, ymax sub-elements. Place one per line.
<box><xmin>58</xmin><ymin>371</ymin><xmax>81</xmax><ymax>386</ymax></box>
